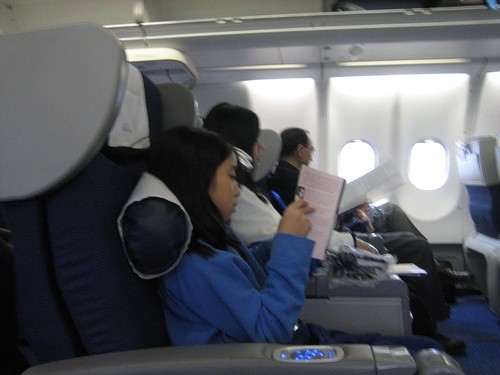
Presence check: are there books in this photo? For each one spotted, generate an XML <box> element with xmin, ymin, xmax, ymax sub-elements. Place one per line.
<box><xmin>294</xmin><ymin>165</ymin><xmax>345</xmax><ymax>264</ymax></box>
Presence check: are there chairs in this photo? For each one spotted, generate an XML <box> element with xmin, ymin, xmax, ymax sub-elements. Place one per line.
<box><xmin>0</xmin><ymin>24</ymin><xmax>500</xmax><ymax>375</ymax></box>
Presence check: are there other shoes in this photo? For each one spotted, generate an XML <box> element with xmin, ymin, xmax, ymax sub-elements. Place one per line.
<box><xmin>453</xmin><ymin>284</ymin><xmax>481</xmax><ymax>296</ymax></box>
<box><xmin>443</xmin><ymin>268</ymin><xmax>472</xmax><ymax>287</ymax></box>
<box><xmin>436</xmin><ymin>332</ymin><xmax>466</xmax><ymax>355</ymax></box>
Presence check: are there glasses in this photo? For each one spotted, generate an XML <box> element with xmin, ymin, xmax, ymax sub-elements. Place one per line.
<box><xmin>301</xmin><ymin>144</ymin><xmax>315</xmax><ymax>154</ymax></box>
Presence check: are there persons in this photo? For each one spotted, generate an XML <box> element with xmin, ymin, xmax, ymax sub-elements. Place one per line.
<box><xmin>159</xmin><ymin>128</ymin><xmax>446</xmax><ymax>355</ymax></box>
<box><xmin>203</xmin><ymin>102</ymin><xmax>466</xmax><ymax>356</ymax></box>
<box><xmin>269</xmin><ymin>128</ymin><xmax>471</xmax><ymax>289</ymax></box>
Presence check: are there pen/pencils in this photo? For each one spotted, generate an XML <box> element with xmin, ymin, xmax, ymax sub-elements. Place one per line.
<box><xmin>269</xmin><ymin>190</ymin><xmax>288</xmax><ymax>211</ymax></box>
